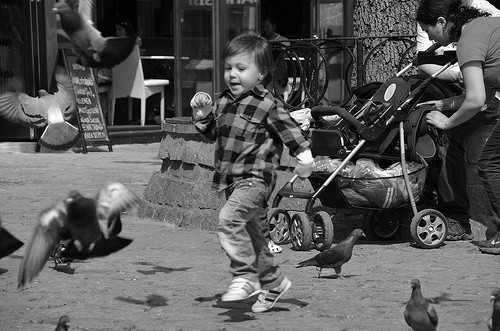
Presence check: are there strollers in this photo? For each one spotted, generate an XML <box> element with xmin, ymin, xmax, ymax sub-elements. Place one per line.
<box><xmin>266</xmin><ymin>40</ymin><xmax>459</xmax><ymax>252</ymax></box>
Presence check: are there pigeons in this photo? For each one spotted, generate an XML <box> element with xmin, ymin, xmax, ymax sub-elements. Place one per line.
<box><xmin>55</xmin><ymin>315</ymin><xmax>70</xmax><ymax>331</ymax></box>
<box><xmin>17</xmin><ymin>183</ymin><xmax>142</xmax><ymax>289</ymax></box>
<box><xmin>51</xmin><ymin>0</ymin><xmax>136</xmax><ymax>68</ymax></box>
<box><xmin>0</xmin><ymin>68</ymin><xmax>81</xmax><ymax>151</ymax></box>
<box><xmin>403</xmin><ymin>279</ymin><xmax>438</xmax><ymax>331</ymax></box>
<box><xmin>485</xmin><ymin>291</ymin><xmax>500</xmax><ymax>331</ymax></box>
<box><xmin>294</xmin><ymin>228</ymin><xmax>366</xmax><ymax>279</ymax></box>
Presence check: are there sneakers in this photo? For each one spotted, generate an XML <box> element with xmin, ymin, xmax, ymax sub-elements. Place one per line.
<box><xmin>478</xmin><ymin>231</ymin><xmax>500</xmax><ymax>255</ymax></box>
<box><xmin>221</xmin><ymin>279</ymin><xmax>261</xmax><ymax>302</ymax></box>
<box><xmin>444</xmin><ymin>219</ymin><xmax>474</xmax><ymax>240</ymax></box>
<box><xmin>251</xmin><ymin>277</ymin><xmax>292</xmax><ymax>312</ymax></box>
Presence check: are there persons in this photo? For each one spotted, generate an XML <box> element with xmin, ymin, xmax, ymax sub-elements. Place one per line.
<box><xmin>415</xmin><ymin>1</ymin><xmax>500</xmax><ymax>255</ymax></box>
<box><xmin>190</xmin><ymin>32</ymin><xmax>315</xmax><ymax>313</ymax></box>
<box><xmin>261</xmin><ymin>19</ymin><xmax>291</xmax><ymax>55</ymax></box>
<box><xmin>115</xmin><ymin>18</ymin><xmax>132</xmax><ymax>37</ymax></box>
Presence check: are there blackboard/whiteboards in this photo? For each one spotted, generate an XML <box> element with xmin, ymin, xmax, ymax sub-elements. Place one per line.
<box><xmin>62</xmin><ymin>48</ymin><xmax>110</xmax><ymax>141</ymax></box>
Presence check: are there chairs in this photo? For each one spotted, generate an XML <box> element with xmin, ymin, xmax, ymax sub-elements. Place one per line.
<box><xmin>98</xmin><ymin>37</ymin><xmax>169</xmax><ymax>125</ymax></box>
<box><xmin>271</xmin><ymin>48</ymin><xmax>307</xmax><ymax>108</ymax></box>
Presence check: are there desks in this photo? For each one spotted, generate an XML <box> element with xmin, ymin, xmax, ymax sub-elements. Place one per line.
<box><xmin>283</xmin><ymin>57</ymin><xmax>306</xmax><ymax>60</ymax></box>
<box><xmin>141</xmin><ymin>56</ymin><xmax>190</xmax><ymax>60</ymax></box>
